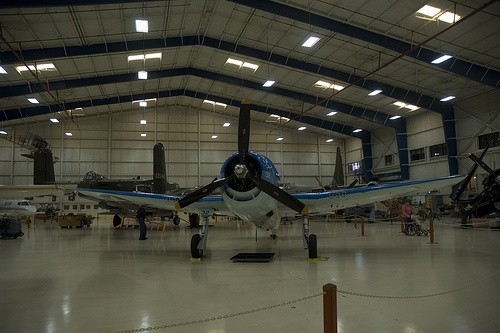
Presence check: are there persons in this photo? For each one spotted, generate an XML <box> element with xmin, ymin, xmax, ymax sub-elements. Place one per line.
<box><xmin>402</xmin><ymin>200</ymin><xmax>414</xmax><ymax>234</ymax></box>
<box><xmin>136</xmin><ymin>204</ymin><xmax>149</xmax><ymax>240</ymax></box>
<box><xmin>411</xmin><ymin>207</ymin><xmax>430</xmax><ymax>232</ymax></box>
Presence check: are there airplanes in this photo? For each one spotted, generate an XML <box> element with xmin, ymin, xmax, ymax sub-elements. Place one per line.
<box><xmin>0</xmin><ymin>199</ymin><xmax>46</xmax><ymax>219</ymax></box>
<box><xmin>74</xmin><ymin>98</ymin><xmax>463</xmax><ymax>259</ymax></box>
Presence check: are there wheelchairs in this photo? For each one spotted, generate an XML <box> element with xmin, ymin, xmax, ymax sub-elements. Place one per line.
<box><xmin>401</xmin><ymin>215</ymin><xmax>429</xmax><ymax>237</ymax></box>
